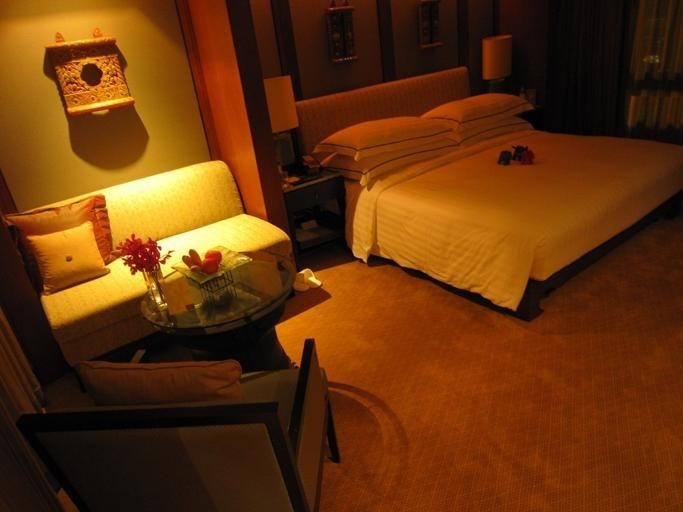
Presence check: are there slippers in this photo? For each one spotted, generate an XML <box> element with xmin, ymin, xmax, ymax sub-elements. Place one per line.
<box><xmin>292</xmin><ymin>272</ymin><xmax>310</xmax><ymax>294</ymax></box>
<box><xmin>299</xmin><ymin>268</ymin><xmax>323</xmax><ymax>290</ymax></box>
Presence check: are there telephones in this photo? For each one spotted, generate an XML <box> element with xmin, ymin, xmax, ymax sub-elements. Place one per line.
<box><xmin>288</xmin><ymin>154</ymin><xmax>324</xmax><ymax>179</ymax></box>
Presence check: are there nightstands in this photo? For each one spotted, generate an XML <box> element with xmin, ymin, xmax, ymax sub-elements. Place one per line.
<box><xmin>278</xmin><ymin>162</ymin><xmax>346</xmax><ymax>255</ymax></box>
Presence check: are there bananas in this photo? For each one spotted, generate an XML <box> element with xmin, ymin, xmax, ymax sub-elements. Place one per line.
<box><xmin>182</xmin><ymin>249</ymin><xmax>202</xmax><ymax>272</ymax></box>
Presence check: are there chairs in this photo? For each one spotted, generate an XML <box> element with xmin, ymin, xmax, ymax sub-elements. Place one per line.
<box><xmin>16</xmin><ymin>338</ymin><xmax>341</xmax><ymax>512</ymax></box>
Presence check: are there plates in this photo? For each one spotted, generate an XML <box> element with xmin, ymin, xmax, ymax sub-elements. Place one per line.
<box><xmin>171</xmin><ymin>245</ymin><xmax>252</xmax><ymax>284</ymax></box>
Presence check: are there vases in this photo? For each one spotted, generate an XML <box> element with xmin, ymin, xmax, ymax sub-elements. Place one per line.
<box><xmin>142</xmin><ymin>263</ymin><xmax>168</xmax><ymax>312</ymax></box>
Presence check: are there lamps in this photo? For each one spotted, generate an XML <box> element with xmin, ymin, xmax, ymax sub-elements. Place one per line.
<box><xmin>264</xmin><ymin>74</ymin><xmax>299</xmax><ymax>181</ymax></box>
<box><xmin>482</xmin><ymin>34</ymin><xmax>516</xmax><ymax>81</ymax></box>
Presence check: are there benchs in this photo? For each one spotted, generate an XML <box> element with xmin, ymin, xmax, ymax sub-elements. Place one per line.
<box><xmin>18</xmin><ymin>160</ymin><xmax>296</xmax><ymax>369</ymax></box>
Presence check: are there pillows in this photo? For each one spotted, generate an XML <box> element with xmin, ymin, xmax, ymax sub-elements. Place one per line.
<box><xmin>80</xmin><ymin>359</ymin><xmax>245</xmax><ymax>404</ymax></box>
<box><xmin>1</xmin><ymin>195</ymin><xmax>122</xmax><ymax>295</ymax></box>
<box><xmin>312</xmin><ymin>93</ymin><xmax>537</xmax><ymax>187</ymax></box>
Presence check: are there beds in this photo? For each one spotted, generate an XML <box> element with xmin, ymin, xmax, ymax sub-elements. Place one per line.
<box><xmin>296</xmin><ymin>66</ymin><xmax>682</xmax><ymax>323</ymax></box>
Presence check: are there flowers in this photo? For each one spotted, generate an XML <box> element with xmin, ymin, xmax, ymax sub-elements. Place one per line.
<box><xmin>110</xmin><ymin>232</ymin><xmax>176</xmax><ymax>304</ymax></box>
